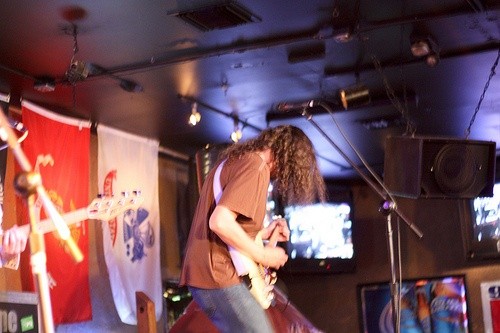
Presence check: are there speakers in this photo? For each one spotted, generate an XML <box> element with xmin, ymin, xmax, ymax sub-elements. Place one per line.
<box><xmin>383</xmin><ymin>136</ymin><xmax>496</xmax><ymax>198</ymax></box>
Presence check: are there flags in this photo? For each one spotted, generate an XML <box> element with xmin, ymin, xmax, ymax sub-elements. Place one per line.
<box><xmin>96</xmin><ymin>120</ymin><xmax>164</xmax><ymax>327</ymax></box>
<box><xmin>13</xmin><ymin>99</ymin><xmax>94</xmax><ymax>328</ymax></box>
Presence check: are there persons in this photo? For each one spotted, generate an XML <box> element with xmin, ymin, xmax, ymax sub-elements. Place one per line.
<box><xmin>178</xmin><ymin>122</ymin><xmax>326</xmax><ymax>333</ymax></box>
<box><xmin>0</xmin><ymin>205</ymin><xmax>29</xmax><ymax>273</ymax></box>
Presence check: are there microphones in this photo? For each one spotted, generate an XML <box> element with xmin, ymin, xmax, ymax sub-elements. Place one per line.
<box><xmin>273</xmin><ymin>100</ymin><xmax>318</xmax><ymax>114</ymax></box>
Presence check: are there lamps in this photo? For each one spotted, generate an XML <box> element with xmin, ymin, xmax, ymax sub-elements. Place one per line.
<box><xmin>285</xmin><ymin>38</ymin><xmax>330</xmax><ymax>65</ymax></box>
<box><xmin>338</xmin><ymin>73</ymin><xmax>373</xmax><ymax>111</ymax></box>
<box><xmin>409</xmin><ymin>28</ymin><xmax>441</xmax><ymax>68</ymax></box>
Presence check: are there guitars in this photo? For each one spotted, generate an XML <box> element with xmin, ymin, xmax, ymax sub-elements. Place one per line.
<box><xmin>226</xmin><ymin>214</ymin><xmax>285</xmax><ymax>310</ymax></box>
<box><xmin>0</xmin><ymin>186</ymin><xmax>143</xmax><ymax>263</ymax></box>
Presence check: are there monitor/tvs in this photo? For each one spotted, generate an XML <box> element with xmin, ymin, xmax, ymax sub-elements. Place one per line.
<box><xmin>457</xmin><ymin>177</ymin><xmax>500</xmax><ymax>259</ymax></box>
<box><xmin>274</xmin><ymin>193</ymin><xmax>356</xmax><ymax>274</ymax></box>
